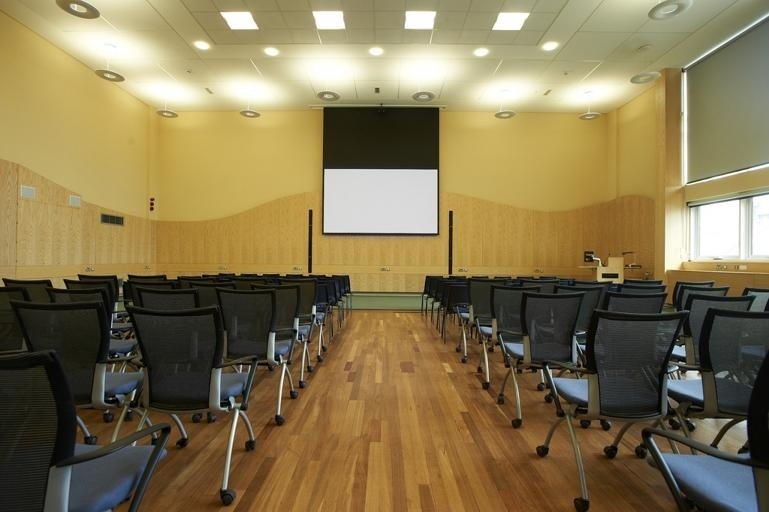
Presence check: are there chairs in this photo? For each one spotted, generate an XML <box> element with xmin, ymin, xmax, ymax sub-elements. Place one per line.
<box><xmin>125</xmin><ymin>304</ymin><xmax>259</xmax><ymax>504</ymax></box>
<box><xmin>576</xmin><ymin>291</ymin><xmax>680</xmax><ymax>431</ymax></box>
<box><xmin>10</xmin><ymin>300</ymin><xmax>158</xmax><ymax>447</ymax></box>
<box><xmin>537</xmin><ymin>308</ymin><xmax>690</xmax><ymax>511</ymax></box>
<box><xmin>215</xmin><ymin>286</ymin><xmax>298</xmax><ymax>426</ymax></box>
<box><xmin>250</xmin><ymin>282</ymin><xmax>313</xmax><ymax>388</ymax></box>
<box><xmin>642</xmin><ymin>347</ymin><xmax>769</xmax><ymax>512</ymax></box>
<box><xmin>635</xmin><ymin>308</ymin><xmax>768</xmax><ymax>458</ymax></box>
<box><xmin>497</xmin><ymin>291</ymin><xmax>590</xmax><ymax>429</ymax></box>
<box><xmin>0</xmin><ymin>349</ymin><xmax>172</xmax><ymax>511</ymax></box>
<box><xmin>669</xmin><ymin>293</ymin><xmax>757</xmax><ymax>431</ymax></box>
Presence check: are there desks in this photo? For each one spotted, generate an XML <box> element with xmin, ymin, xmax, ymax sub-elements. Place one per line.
<box><xmin>578</xmin><ymin>256</ymin><xmax>641</xmax><ymax>283</ymax></box>
